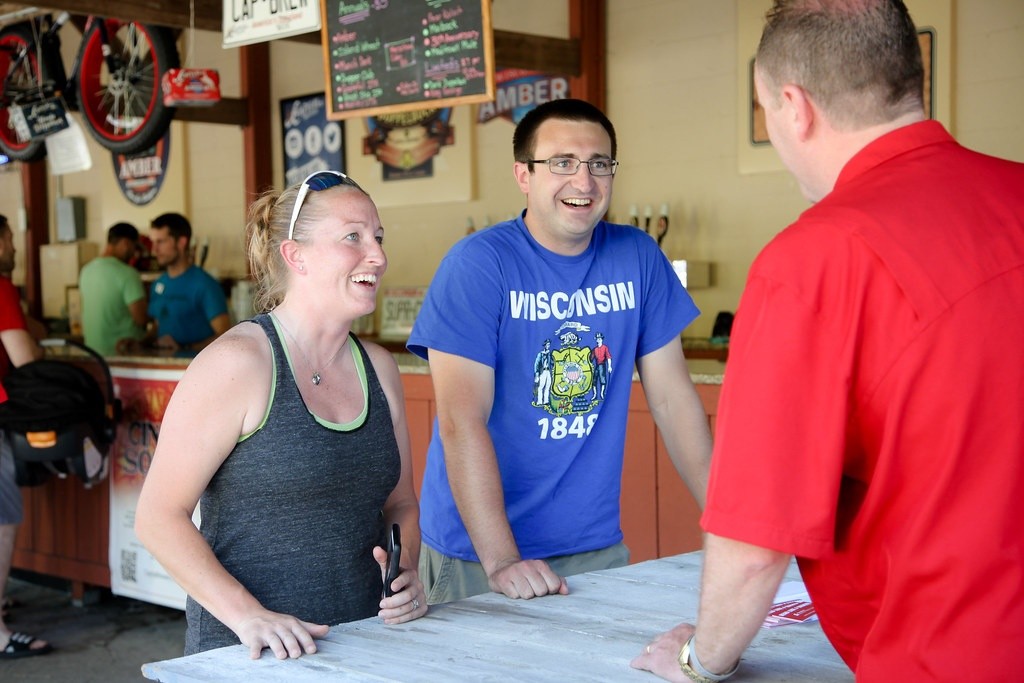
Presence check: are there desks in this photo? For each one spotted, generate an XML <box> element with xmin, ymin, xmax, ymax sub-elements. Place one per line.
<box><xmin>140</xmin><ymin>550</ymin><xmax>857</xmax><ymax>683</ymax></box>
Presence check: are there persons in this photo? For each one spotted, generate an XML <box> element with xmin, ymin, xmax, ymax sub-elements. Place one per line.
<box><xmin>135</xmin><ymin>169</ymin><xmax>429</xmax><ymax>660</ymax></box>
<box><xmin>78</xmin><ymin>221</ymin><xmax>147</xmax><ymax>358</ymax></box>
<box><xmin>629</xmin><ymin>0</ymin><xmax>1024</xmax><ymax>683</ymax></box>
<box><xmin>0</xmin><ymin>213</ymin><xmax>52</xmax><ymax>661</ymax></box>
<box><xmin>116</xmin><ymin>213</ymin><xmax>232</xmax><ymax>358</ymax></box>
<box><xmin>405</xmin><ymin>98</ymin><xmax>714</xmax><ymax>606</ymax></box>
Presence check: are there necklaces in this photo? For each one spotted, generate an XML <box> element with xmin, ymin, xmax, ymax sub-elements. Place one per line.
<box><xmin>271</xmin><ymin>309</ymin><xmax>350</xmax><ymax>386</ymax></box>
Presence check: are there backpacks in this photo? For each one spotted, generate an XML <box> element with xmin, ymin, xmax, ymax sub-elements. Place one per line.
<box><xmin>1</xmin><ymin>338</ymin><xmax>124</xmax><ymax>490</ymax></box>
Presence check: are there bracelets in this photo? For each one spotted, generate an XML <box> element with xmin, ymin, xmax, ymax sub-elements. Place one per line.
<box><xmin>690</xmin><ymin>636</ymin><xmax>741</xmax><ymax>680</ymax></box>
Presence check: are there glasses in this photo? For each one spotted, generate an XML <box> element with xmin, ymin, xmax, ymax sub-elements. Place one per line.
<box><xmin>521</xmin><ymin>156</ymin><xmax>619</xmax><ymax>176</ymax></box>
<box><xmin>288</xmin><ymin>170</ymin><xmax>368</xmax><ymax>240</ymax></box>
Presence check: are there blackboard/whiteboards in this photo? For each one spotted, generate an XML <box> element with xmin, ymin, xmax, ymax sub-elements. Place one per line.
<box><xmin>319</xmin><ymin>0</ymin><xmax>497</xmax><ymax>121</ymax></box>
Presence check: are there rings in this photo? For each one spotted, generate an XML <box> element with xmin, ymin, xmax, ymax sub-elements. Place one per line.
<box><xmin>645</xmin><ymin>640</ymin><xmax>657</xmax><ymax>654</ymax></box>
<box><xmin>411</xmin><ymin>598</ymin><xmax>419</xmax><ymax>611</ymax></box>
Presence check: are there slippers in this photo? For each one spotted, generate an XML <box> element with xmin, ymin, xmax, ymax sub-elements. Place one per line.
<box><xmin>0</xmin><ymin>631</ymin><xmax>54</xmax><ymax>659</ymax></box>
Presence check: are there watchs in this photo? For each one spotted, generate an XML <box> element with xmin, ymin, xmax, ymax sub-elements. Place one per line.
<box><xmin>677</xmin><ymin>633</ymin><xmax>735</xmax><ymax>683</ymax></box>
